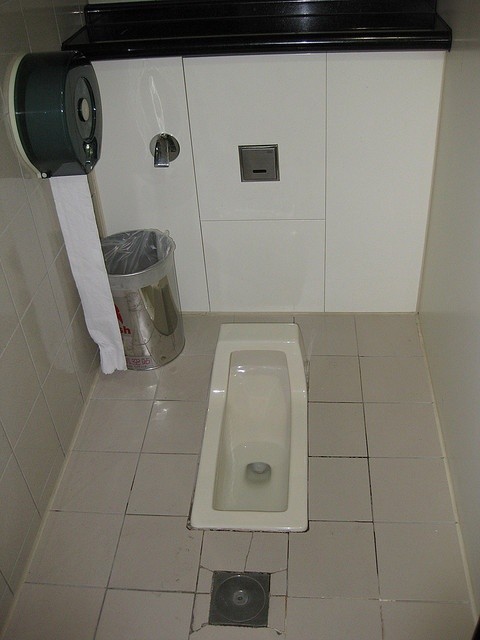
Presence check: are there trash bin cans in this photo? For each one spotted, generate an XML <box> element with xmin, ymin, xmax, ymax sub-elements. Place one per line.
<box><xmin>100</xmin><ymin>228</ymin><xmax>185</xmax><ymax>371</ymax></box>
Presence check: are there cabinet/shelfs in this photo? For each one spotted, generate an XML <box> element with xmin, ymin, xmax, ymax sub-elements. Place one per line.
<box><xmin>89</xmin><ymin>50</ymin><xmax>448</xmax><ymax>313</ymax></box>
